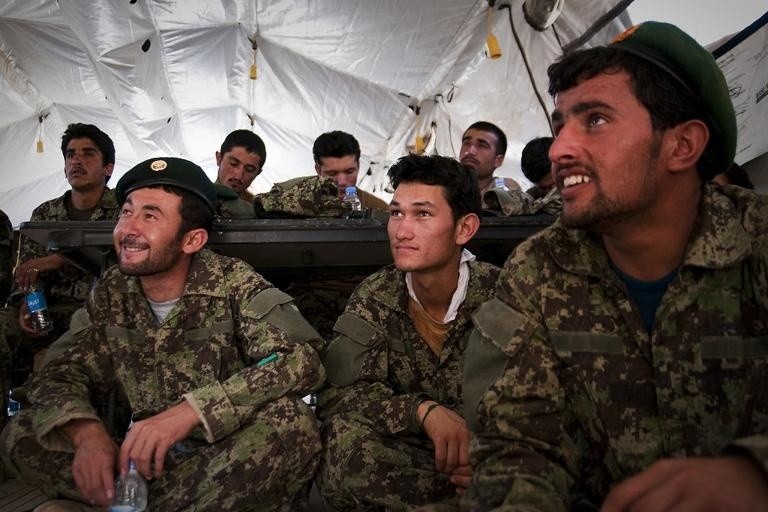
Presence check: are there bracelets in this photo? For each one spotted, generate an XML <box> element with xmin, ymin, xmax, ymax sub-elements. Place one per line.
<box><xmin>421</xmin><ymin>403</ymin><xmax>444</xmax><ymax>428</ymax></box>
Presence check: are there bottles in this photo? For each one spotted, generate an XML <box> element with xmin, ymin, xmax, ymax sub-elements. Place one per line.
<box><xmin>495</xmin><ymin>178</ymin><xmax>509</xmax><ymax>191</ymax></box>
<box><xmin>107</xmin><ymin>459</ymin><xmax>148</xmax><ymax>512</ymax></box>
<box><xmin>9</xmin><ymin>398</ymin><xmax>18</xmax><ymax>416</ymax></box>
<box><xmin>342</xmin><ymin>187</ymin><xmax>361</xmax><ymax>211</ymax></box>
<box><xmin>25</xmin><ymin>281</ymin><xmax>50</xmax><ymax>331</ymax></box>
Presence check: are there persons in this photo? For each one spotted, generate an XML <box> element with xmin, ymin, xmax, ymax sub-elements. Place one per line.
<box><xmin>211</xmin><ymin>130</ymin><xmax>266</xmax><ymax>220</ymax></box>
<box><xmin>313</xmin><ymin>152</ymin><xmax>504</xmax><ymax>511</ymax></box>
<box><xmin>460</xmin><ymin>122</ymin><xmax>523</xmax><ymax>217</ymax></box>
<box><xmin>255</xmin><ymin>131</ymin><xmax>393</xmax><ymax>343</ymax></box>
<box><xmin>0</xmin><ymin>123</ymin><xmax>121</xmax><ymax>432</ymax></box>
<box><xmin>0</xmin><ymin>211</ymin><xmax>20</xmax><ymax>309</ymax></box>
<box><xmin>460</xmin><ymin>20</ymin><xmax>767</xmax><ymax>512</ymax></box>
<box><xmin>504</xmin><ymin>137</ymin><xmax>564</xmax><ymax>216</ymax></box>
<box><xmin>712</xmin><ymin>162</ymin><xmax>754</xmax><ymax>190</ymax></box>
<box><xmin>0</xmin><ymin>157</ymin><xmax>328</xmax><ymax>512</ymax></box>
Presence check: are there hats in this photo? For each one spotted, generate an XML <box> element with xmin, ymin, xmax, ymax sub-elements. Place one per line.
<box><xmin>116</xmin><ymin>157</ymin><xmax>217</xmax><ymax>214</ymax></box>
<box><xmin>607</xmin><ymin>20</ymin><xmax>735</xmax><ymax>176</ymax></box>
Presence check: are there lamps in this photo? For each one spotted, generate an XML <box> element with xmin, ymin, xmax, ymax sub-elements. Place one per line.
<box><xmin>250</xmin><ymin>43</ymin><xmax>257</xmax><ymax>79</ymax></box>
<box><xmin>416</xmin><ymin>115</ymin><xmax>423</xmax><ymax>152</ymax></box>
<box><xmin>37</xmin><ymin>116</ymin><xmax>44</xmax><ymax>153</ymax></box>
<box><xmin>487</xmin><ymin>7</ymin><xmax>502</xmax><ymax>58</ymax></box>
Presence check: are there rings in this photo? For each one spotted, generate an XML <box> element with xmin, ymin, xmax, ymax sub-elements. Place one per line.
<box><xmin>33</xmin><ymin>268</ymin><xmax>40</xmax><ymax>273</ymax></box>
<box><xmin>26</xmin><ymin>268</ymin><xmax>33</xmax><ymax>273</ymax></box>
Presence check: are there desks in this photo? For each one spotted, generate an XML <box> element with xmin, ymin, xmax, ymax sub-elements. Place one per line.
<box><xmin>19</xmin><ymin>214</ymin><xmax>558</xmax><ymax>266</ymax></box>
